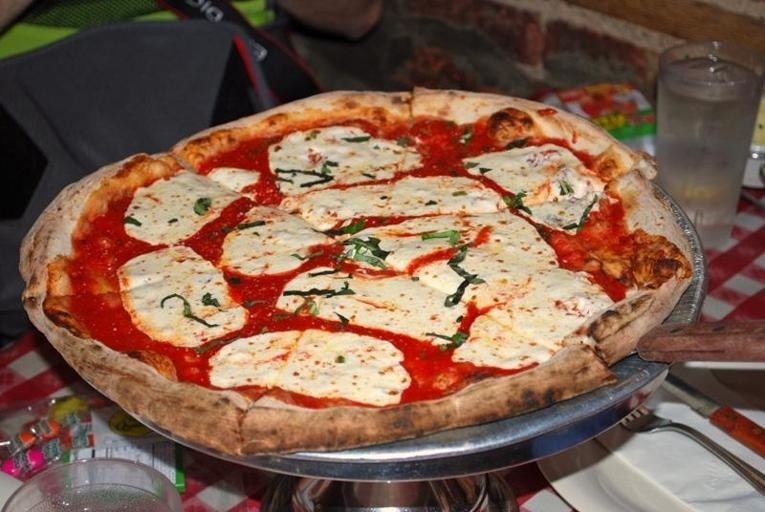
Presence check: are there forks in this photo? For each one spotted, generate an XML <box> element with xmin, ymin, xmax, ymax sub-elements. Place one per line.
<box><xmin>620</xmin><ymin>407</ymin><xmax>765</xmax><ymax>501</ymax></box>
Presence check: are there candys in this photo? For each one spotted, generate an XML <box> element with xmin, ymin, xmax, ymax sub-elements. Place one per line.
<box><xmin>0</xmin><ymin>393</ymin><xmax>107</xmax><ymax>483</ymax></box>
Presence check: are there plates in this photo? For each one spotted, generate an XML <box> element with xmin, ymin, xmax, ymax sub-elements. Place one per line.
<box><xmin>147</xmin><ymin>182</ymin><xmax>708</xmax><ymax>482</ymax></box>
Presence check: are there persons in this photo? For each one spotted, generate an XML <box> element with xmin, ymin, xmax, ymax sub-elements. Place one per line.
<box><xmin>1</xmin><ymin>0</ymin><xmax>385</xmax><ymax>41</ymax></box>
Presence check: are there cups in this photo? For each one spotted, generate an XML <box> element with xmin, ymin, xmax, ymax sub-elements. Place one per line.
<box><xmin>655</xmin><ymin>40</ymin><xmax>762</xmax><ymax>250</ymax></box>
<box><xmin>0</xmin><ymin>458</ymin><xmax>183</xmax><ymax>512</ymax></box>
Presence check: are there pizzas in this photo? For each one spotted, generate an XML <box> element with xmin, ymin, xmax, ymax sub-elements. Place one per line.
<box><xmin>17</xmin><ymin>83</ymin><xmax>694</xmax><ymax>457</ymax></box>
